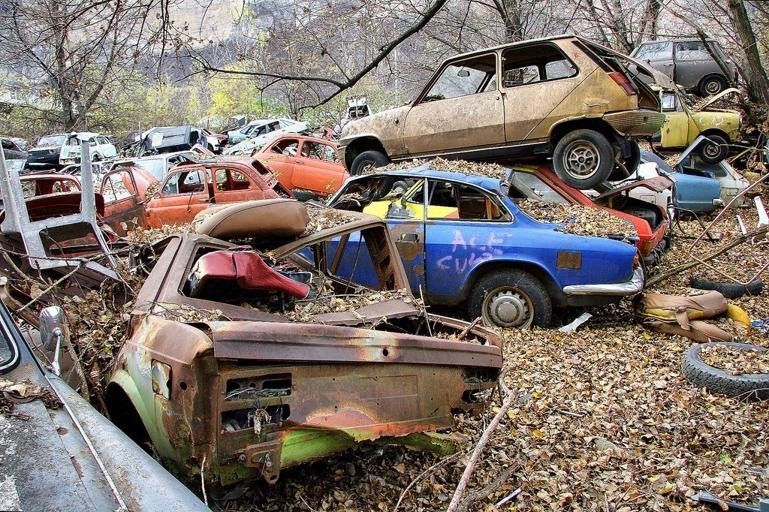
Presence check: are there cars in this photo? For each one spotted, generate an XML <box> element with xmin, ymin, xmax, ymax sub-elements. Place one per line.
<box><xmin>295</xmin><ymin>166</ymin><xmax>647</xmax><ymax>329</ymax></box>
<box><xmin>0</xmin><ymin>34</ymin><xmax>750</xmax><ymax>511</ymax></box>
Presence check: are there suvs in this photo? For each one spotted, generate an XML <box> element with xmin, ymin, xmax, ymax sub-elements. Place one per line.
<box><xmin>628</xmin><ymin>38</ymin><xmax>736</xmax><ymax>98</ymax></box>
